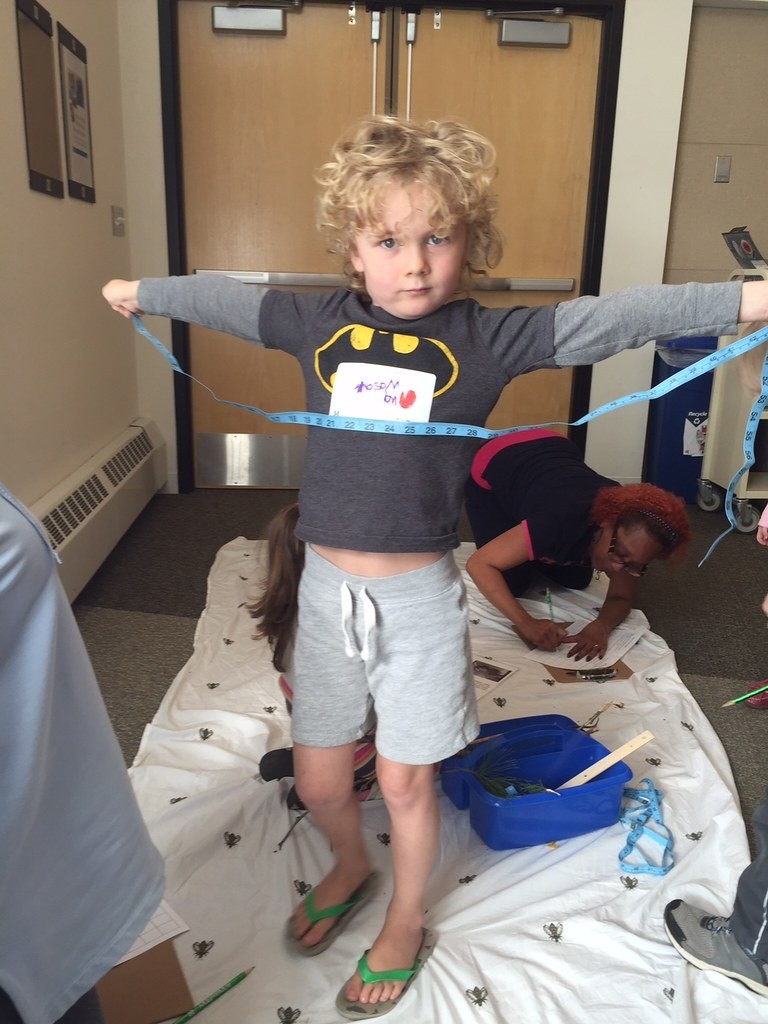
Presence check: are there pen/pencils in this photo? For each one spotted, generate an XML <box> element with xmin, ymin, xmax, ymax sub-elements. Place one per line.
<box><xmin>566</xmin><ymin>668</ymin><xmax>619</xmax><ymax>675</ymax></box>
<box><xmin>546</xmin><ymin>587</ymin><xmax>560</xmax><ymax>653</ymax></box>
<box><xmin>721</xmin><ymin>686</ymin><xmax>768</xmax><ymax>708</ymax></box>
<box><xmin>172</xmin><ymin>966</ymin><xmax>256</xmax><ymax>1024</ymax></box>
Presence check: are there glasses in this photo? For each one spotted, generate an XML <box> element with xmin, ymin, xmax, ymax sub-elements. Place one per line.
<box><xmin>608</xmin><ymin>520</ymin><xmax>647</xmax><ymax>577</ymax></box>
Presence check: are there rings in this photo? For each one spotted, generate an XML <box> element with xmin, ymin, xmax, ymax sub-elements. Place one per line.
<box><xmin>593</xmin><ymin>645</ymin><xmax>598</xmax><ymax>648</ymax></box>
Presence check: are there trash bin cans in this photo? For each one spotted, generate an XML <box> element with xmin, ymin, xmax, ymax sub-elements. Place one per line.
<box><xmin>645</xmin><ymin>337</ymin><xmax>718</xmax><ymax>503</ymax></box>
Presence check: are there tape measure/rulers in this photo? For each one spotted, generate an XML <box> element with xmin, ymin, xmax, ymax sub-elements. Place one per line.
<box><xmin>555</xmin><ymin>730</ymin><xmax>655</xmax><ymax>790</ymax></box>
<box><xmin>131</xmin><ymin>313</ymin><xmax>768</xmax><ymax>568</ymax></box>
<box><xmin>618</xmin><ymin>778</ymin><xmax>675</xmax><ymax>876</ymax></box>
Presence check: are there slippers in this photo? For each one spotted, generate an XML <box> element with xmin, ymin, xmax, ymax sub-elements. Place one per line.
<box><xmin>334</xmin><ymin>927</ymin><xmax>435</xmax><ymax>1021</ymax></box>
<box><xmin>285</xmin><ymin>871</ymin><xmax>379</xmax><ymax>958</ymax></box>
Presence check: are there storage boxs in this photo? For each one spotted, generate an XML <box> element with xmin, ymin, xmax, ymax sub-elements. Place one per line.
<box><xmin>461</xmin><ymin>729</ymin><xmax>632</xmax><ymax>851</ymax></box>
<box><xmin>440</xmin><ymin>714</ymin><xmax>580</xmax><ymax>809</ymax></box>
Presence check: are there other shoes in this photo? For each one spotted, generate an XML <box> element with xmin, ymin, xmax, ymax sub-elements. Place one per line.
<box><xmin>744</xmin><ymin>679</ymin><xmax>767</xmax><ymax>708</ymax></box>
<box><xmin>260</xmin><ymin>747</ymin><xmax>294</xmax><ymax>782</ymax></box>
<box><xmin>287</xmin><ymin>785</ymin><xmax>307</xmax><ymax>810</ymax></box>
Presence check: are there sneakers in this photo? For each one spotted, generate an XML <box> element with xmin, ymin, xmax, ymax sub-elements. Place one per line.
<box><xmin>663</xmin><ymin>899</ymin><xmax>768</xmax><ymax>996</ymax></box>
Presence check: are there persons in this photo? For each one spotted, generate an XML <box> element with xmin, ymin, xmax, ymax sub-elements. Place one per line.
<box><xmin>664</xmin><ymin>785</ymin><xmax>768</xmax><ymax>996</ymax></box>
<box><xmin>247</xmin><ymin>500</ymin><xmax>442</xmax><ymax>810</ymax></box>
<box><xmin>757</xmin><ymin>504</ymin><xmax>768</xmax><ymax>617</ymax></box>
<box><xmin>465</xmin><ymin>428</ymin><xmax>688</xmax><ymax>662</ymax></box>
<box><xmin>0</xmin><ymin>485</ymin><xmax>165</xmax><ymax>1024</ymax></box>
<box><xmin>101</xmin><ymin>112</ymin><xmax>768</xmax><ymax>1020</ymax></box>
